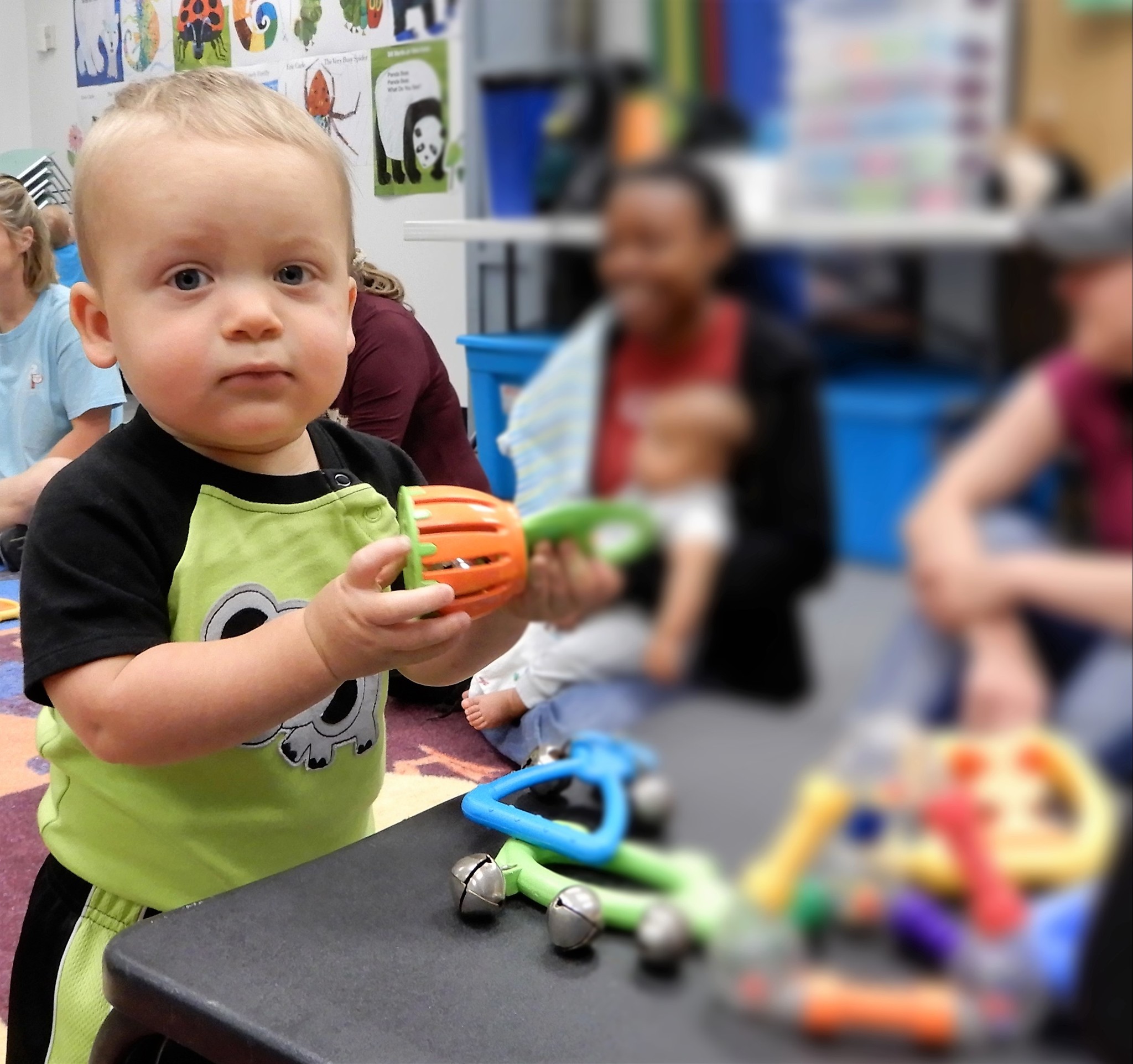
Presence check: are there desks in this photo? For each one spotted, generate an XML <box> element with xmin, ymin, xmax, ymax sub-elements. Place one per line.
<box><xmin>454</xmin><ymin>323</ymin><xmax>580</xmax><ymax>510</ymax></box>
<box><xmin>84</xmin><ymin>698</ymin><xmax>1133</xmax><ymax>1064</ymax></box>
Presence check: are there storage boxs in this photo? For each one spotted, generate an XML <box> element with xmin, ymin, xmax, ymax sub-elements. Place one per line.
<box><xmin>811</xmin><ymin>372</ymin><xmax>1076</xmax><ymax>572</ymax></box>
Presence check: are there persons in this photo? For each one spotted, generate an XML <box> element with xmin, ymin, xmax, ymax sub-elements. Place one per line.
<box><xmin>318</xmin><ymin>241</ymin><xmax>496</xmax><ymax>712</ymax></box>
<box><xmin>1</xmin><ymin>84</ymin><xmax>629</xmax><ymax>1062</ymax></box>
<box><xmin>899</xmin><ymin>175</ymin><xmax>1130</xmax><ymax>786</ymax></box>
<box><xmin>0</xmin><ymin>170</ymin><xmax>128</xmax><ymax>574</ymax></box>
<box><xmin>504</xmin><ymin>157</ymin><xmax>845</xmax><ymax>709</ymax></box>
<box><xmin>465</xmin><ymin>384</ymin><xmax>760</xmax><ymax>727</ymax></box>
